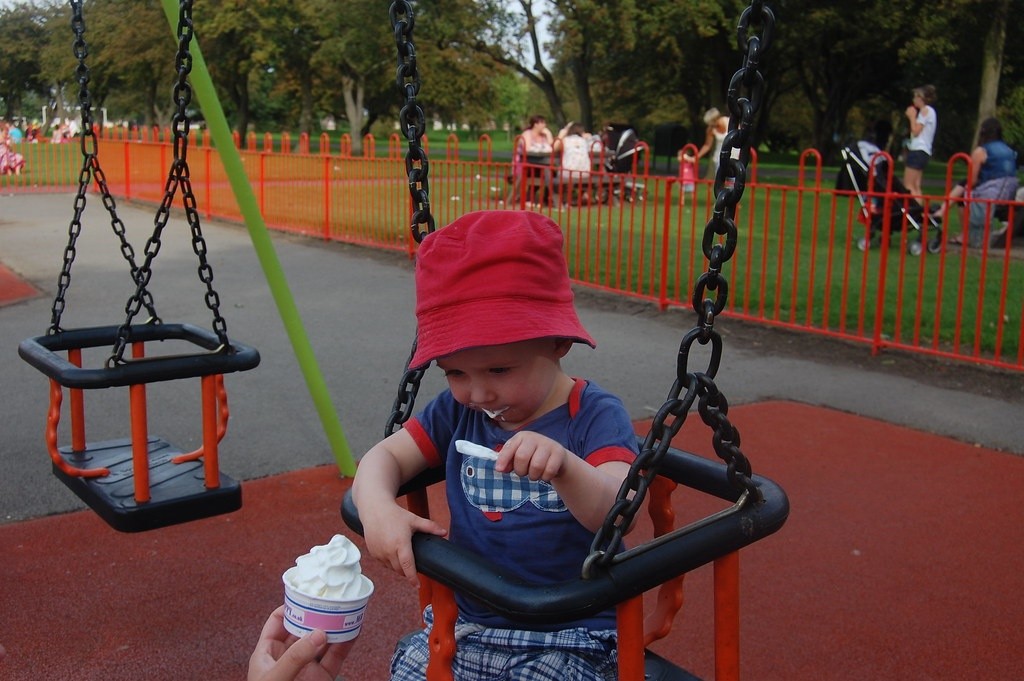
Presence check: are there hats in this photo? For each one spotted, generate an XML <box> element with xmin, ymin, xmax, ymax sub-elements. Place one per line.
<box><xmin>408</xmin><ymin>209</ymin><xmax>596</xmax><ymax>371</ymax></box>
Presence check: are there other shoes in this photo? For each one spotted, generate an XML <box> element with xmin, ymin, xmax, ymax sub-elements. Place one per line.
<box><xmin>922</xmin><ymin>212</ymin><xmax>942</xmax><ymax>220</ymax></box>
<box><xmin>949</xmin><ymin>237</ymin><xmax>962</xmax><ymax>244</ymax></box>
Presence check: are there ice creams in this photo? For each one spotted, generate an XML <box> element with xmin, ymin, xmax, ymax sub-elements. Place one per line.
<box><xmin>281</xmin><ymin>534</ymin><xmax>374</xmax><ymax>643</ymax></box>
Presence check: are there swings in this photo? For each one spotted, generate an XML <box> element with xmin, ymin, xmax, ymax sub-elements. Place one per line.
<box><xmin>15</xmin><ymin>0</ymin><xmax>264</xmax><ymax>534</ymax></box>
<box><xmin>382</xmin><ymin>0</ymin><xmax>792</xmax><ymax>681</ymax></box>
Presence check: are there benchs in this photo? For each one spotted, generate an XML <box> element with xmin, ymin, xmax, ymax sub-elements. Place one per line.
<box><xmin>507</xmin><ymin>176</ymin><xmax>627</xmax><ymax>185</ymax></box>
<box><xmin>968</xmin><ymin>205</ymin><xmax>1024</xmax><ymax>248</ymax></box>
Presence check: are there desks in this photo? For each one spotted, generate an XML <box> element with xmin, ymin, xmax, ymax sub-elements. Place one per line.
<box><xmin>506</xmin><ymin>157</ymin><xmax>620</xmax><ymax>209</ymax></box>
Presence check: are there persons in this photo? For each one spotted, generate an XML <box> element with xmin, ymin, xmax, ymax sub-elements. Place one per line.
<box><xmin>0</xmin><ymin>115</ymin><xmax>81</xmax><ymax>175</ymax></box>
<box><xmin>350</xmin><ymin>210</ymin><xmax>643</xmax><ymax>681</ymax></box>
<box><xmin>510</xmin><ymin>115</ymin><xmax>617</xmax><ymax>210</ymax></box>
<box><xmin>922</xmin><ymin>116</ymin><xmax>1017</xmax><ymax>246</ymax></box>
<box><xmin>677</xmin><ymin>108</ymin><xmax>744</xmax><ymax>206</ymax></box>
<box><xmin>901</xmin><ymin>85</ymin><xmax>937</xmax><ymax>203</ymax></box>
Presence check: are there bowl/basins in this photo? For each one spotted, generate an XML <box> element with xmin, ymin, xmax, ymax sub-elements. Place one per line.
<box><xmin>281</xmin><ymin>567</ymin><xmax>374</xmax><ymax>643</ymax></box>
